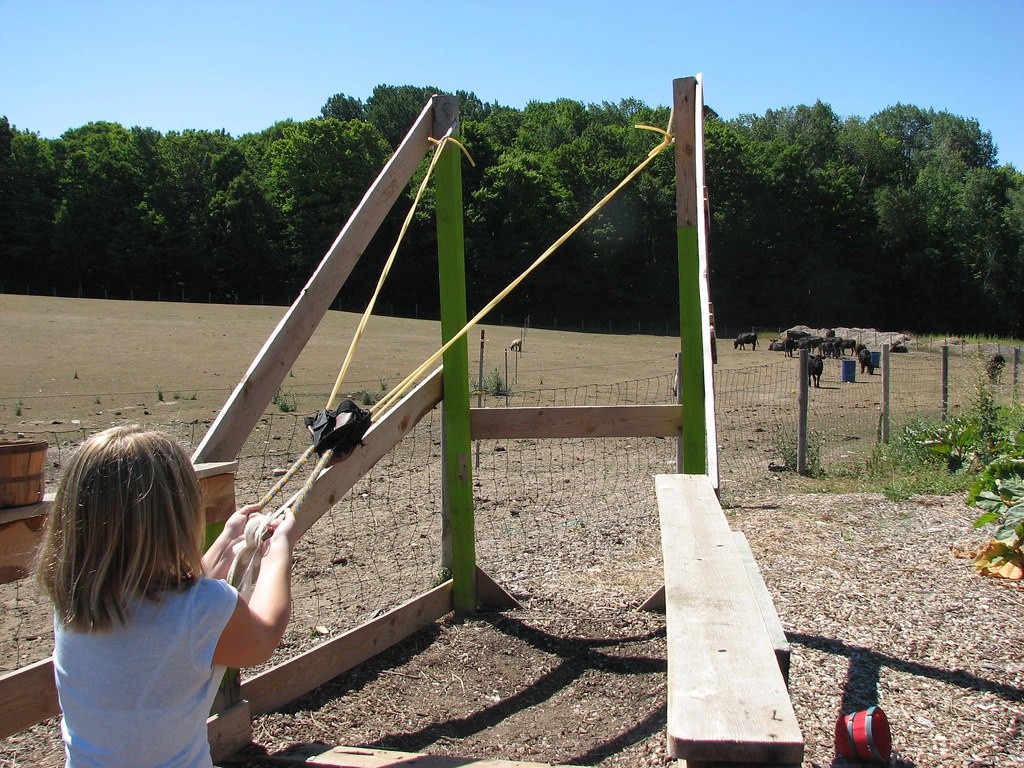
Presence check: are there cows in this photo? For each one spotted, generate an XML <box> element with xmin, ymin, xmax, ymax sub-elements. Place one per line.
<box><xmin>768</xmin><ymin>329</ymin><xmax>909</xmax><ymax>388</ymax></box>
<box><xmin>509</xmin><ymin>339</ymin><xmax>521</xmax><ymax>352</ymax></box>
<box><xmin>985</xmin><ymin>354</ymin><xmax>1005</xmax><ymax>379</ymax></box>
<box><xmin>734</xmin><ymin>332</ymin><xmax>759</xmax><ymax>351</ymax></box>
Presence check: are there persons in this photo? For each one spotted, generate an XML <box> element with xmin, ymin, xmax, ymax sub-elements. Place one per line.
<box><xmin>34</xmin><ymin>424</ymin><xmax>298</xmax><ymax>768</ymax></box>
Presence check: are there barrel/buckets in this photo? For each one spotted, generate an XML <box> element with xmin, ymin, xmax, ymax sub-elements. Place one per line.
<box><xmin>840</xmin><ymin>359</ymin><xmax>856</xmax><ymax>382</ymax></box>
<box><xmin>835</xmin><ymin>706</ymin><xmax>892</xmax><ymax>765</ymax></box>
<box><xmin>0</xmin><ymin>439</ymin><xmax>49</xmax><ymax>508</ymax></box>
<box><xmin>870</xmin><ymin>351</ymin><xmax>881</xmax><ymax>368</ymax></box>
